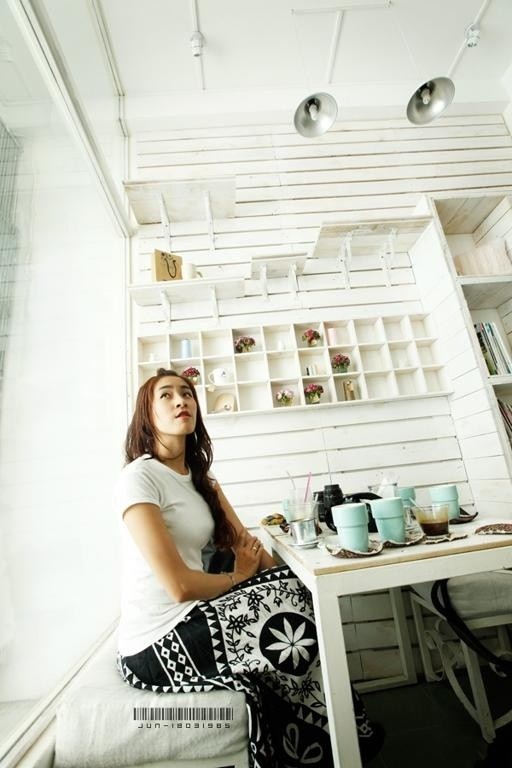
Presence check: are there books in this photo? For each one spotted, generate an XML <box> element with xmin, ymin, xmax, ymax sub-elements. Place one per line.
<box><xmin>474</xmin><ymin>321</ymin><xmax>512</xmax><ymax>450</ymax></box>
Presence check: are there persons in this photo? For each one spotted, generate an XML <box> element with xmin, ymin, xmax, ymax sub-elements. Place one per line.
<box><xmin>115</xmin><ymin>371</ymin><xmax>386</xmax><ymax>768</ymax></box>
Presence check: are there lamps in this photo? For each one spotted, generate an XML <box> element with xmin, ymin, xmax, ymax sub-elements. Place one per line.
<box><xmin>384</xmin><ymin>5</ymin><xmax>456</xmax><ymax>126</ymax></box>
<box><xmin>290</xmin><ymin>9</ymin><xmax>348</xmax><ymax>138</ymax></box>
<box><xmin>189</xmin><ymin>31</ymin><xmax>205</xmax><ymax>57</ymax></box>
<box><xmin>462</xmin><ymin>23</ymin><xmax>482</xmax><ymax>49</ymax></box>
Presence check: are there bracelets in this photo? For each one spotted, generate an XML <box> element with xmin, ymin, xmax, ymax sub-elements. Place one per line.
<box><xmin>222</xmin><ymin>572</ymin><xmax>237</xmax><ymax>585</ymax></box>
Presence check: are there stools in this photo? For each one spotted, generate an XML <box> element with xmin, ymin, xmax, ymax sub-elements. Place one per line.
<box><xmin>52</xmin><ymin>658</ymin><xmax>249</xmax><ymax>768</ymax></box>
<box><xmin>409</xmin><ymin>569</ymin><xmax>512</xmax><ymax>740</ymax></box>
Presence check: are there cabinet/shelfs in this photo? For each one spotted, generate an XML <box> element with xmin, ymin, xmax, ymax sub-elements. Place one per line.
<box><xmin>408</xmin><ymin>190</ymin><xmax>512</xmax><ymax>520</ymax></box>
<box><xmin>135</xmin><ymin>310</ymin><xmax>454</xmax><ymax>418</ymax></box>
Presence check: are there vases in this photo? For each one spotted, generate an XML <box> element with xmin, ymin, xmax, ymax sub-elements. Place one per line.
<box><xmin>310</xmin><ymin>393</ymin><xmax>320</xmax><ymax>403</ymax></box>
<box><xmin>279</xmin><ymin>398</ymin><xmax>288</xmax><ymax>406</ymax></box>
<box><xmin>307</xmin><ymin>339</ymin><xmax>313</xmax><ymax>347</ymax></box>
<box><xmin>241</xmin><ymin>345</ymin><xmax>248</xmax><ymax>352</ymax></box>
<box><xmin>337</xmin><ymin>364</ymin><xmax>348</xmax><ymax>373</ymax></box>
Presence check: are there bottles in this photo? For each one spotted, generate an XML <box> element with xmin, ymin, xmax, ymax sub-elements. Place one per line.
<box><xmin>328</xmin><ymin>328</ymin><xmax>337</xmax><ymax>347</ymax></box>
<box><xmin>343</xmin><ymin>380</ymin><xmax>355</xmax><ymax>401</ymax></box>
<box><xmin>181</xmin><ymin>338</ymin><xmax>192</xmax><ymax>357</ymax></box>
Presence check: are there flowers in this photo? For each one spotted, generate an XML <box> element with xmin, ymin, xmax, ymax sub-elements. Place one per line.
<box><xmin>180</xmin><ymin>367</ymin><xmax>199</xmax><ymax>377</ymax></box>
<box><xmin>275</xmin><ymin>389</ymin><xmax>295</xmax><ymax>401</ymax></box>
<box><xmin>300</xmin><ymin>328</ymin><xmax>320</xmax><ymax>343</ymax></box>
<box><xmin>304</xmin><ymin>384</ymin><xmax>324</xmax><ymax>395</ymax></box>
<box><xmin>330</xmin><ymin>352</ymin><xmax>351</xmax><ymax>369</ymax></box>
<box><xmin>233</xmin><ymin>335</ymin><xmax>256</xmax><ymax>352</ymax></box>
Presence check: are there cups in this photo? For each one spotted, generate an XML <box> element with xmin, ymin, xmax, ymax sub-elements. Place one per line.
<box><xmin>181</xmin><ymin>264</ymin><xmax>195</xmax><ymax>279</ymax></box>
<box><xmin>331</xmin><ymin>484</ymin><xmax>459</xmax><ymax>553</ymax></box>
<box><xmin>207</xmin><ymin>367</ymin><xmax>229</xmax><ymax>386</ymax></box>
<box><xmin>282</xmin><ymin>488</ymin><xmax>319</xmax><ymax>549</ymax></box>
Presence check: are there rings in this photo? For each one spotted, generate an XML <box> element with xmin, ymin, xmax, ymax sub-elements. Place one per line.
<box><xmin>253</xmin><ymin>546</ymin><xmax>257</xmax><ymax>551</ymax></box>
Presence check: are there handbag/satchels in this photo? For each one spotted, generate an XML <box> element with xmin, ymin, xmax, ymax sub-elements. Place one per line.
<box><xmin>153</xmin><ymin>248</ymin><xmax>183</xmax><ymax>281</ymax></box>
